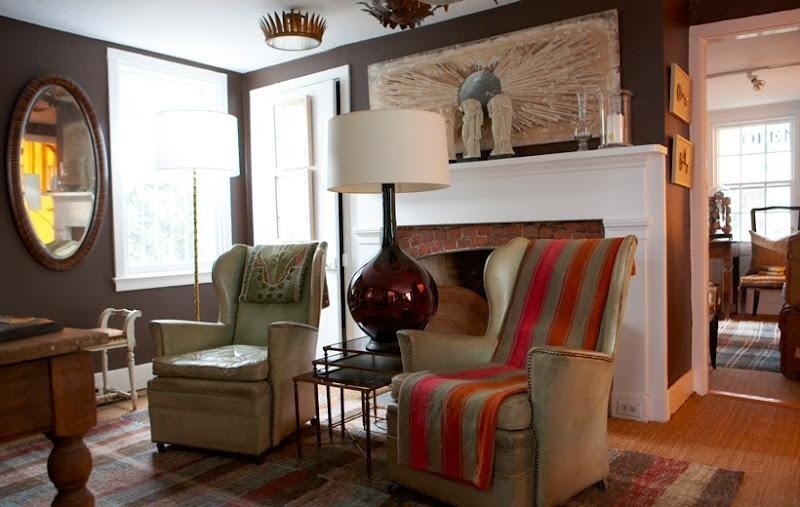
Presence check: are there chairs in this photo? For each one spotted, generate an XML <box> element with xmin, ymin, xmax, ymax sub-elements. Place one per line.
<box><xmin>389</xmin><ymin>234</ymin><xmax>637</xmax><ymax>505</ymax></box>
<box><xmin>740</xmin><ymin>206</ymin><xmax>800</xmax><ymax>319</ymax></box>
<box><xmin>149</xmin><ymin>241</ymin><xmax>329</xmax><ymax>463</ymax></box>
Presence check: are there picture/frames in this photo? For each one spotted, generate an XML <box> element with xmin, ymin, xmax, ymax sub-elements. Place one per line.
<box><xmin>670</xmin><ymin>134</ymin><xmax>694</xmax><ymax>188</ymax></box>
<box><xmin>668</xmin><ymin>64</ymin><xmax>693</xmax><ymax>123</ymax></box>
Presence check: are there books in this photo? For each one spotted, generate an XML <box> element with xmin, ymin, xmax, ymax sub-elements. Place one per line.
<box><xmin>759</xmin><ymin>264</ymin><xmax>786</xmax><ymax>272</ymax></box>
<box><xmin>757</xmin><ymin>270</ymin><xmax>785</xmax><ymax>277</ymax></box>
<box><xmin>0</xmin><ymin>313</ymin><xmax>61</xmax><ymax>342</ymax></box>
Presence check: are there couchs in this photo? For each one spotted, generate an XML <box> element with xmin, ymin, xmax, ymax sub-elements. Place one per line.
<box><xmin>778</xmin><ymin>229</ymin><xmax>800</xmax><ymax>380</ymax></box>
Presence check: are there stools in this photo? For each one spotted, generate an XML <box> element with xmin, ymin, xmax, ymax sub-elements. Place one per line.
<box><xmin>84</xmin><ymin>308</ymin><xmax>142</xmax><ymax>410</ymax></box>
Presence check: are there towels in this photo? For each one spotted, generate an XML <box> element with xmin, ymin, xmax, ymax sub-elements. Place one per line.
<box><xmin>757</xmin><ymin>263</ymin><xmax>786</xmax><ymax>276</ymax></box>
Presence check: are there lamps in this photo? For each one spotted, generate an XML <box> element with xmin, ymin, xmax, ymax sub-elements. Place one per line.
<box><xmin>747</xmin><ymin>71</ymin><xmax>765</xmax><ymax>91</ymax></box>
<box><xmin>258</xmin><ymin>8</ymin><xmax>328</xmax><ymax>50</ymax></box>
<box><xmin>326</xmin><ymin>109</ymin><xmax>451</xmax><ymax>354</ymax></box>
<box><xmin>152</xmin><ymin>112</ymin><xmax>240</xmax><ymax>321</ymax></box>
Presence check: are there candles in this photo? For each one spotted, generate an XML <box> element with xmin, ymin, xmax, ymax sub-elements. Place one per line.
<box><xmin>607</xmin><ymin>113</ymin><xmax>624</xmax><ymax>144</ymax></box>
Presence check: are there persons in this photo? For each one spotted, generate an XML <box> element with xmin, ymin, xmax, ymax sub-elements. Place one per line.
<box><xmin>458</xmin><ymin>97</ymin><xmax>485</xmax><ymax>158</ymax></box>
<box><xmin>487</xmin><ymin>92</ymin><xmax>517</xmax><ymax>155</ymax></box>
<box><xmin>439</xmin><ymin>105</ymin><xmax>458</xmax><ymax>162</ymax></box>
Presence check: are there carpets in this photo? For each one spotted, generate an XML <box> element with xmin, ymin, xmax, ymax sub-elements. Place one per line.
<box><xmin>0</xmin><ymin>406</ymin><xmax>745</xmax><ymax>506</ymax></box>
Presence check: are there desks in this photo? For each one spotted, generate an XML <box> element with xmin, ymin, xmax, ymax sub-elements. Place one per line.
<box><xmin>1</xmin><ymin>327</ymin><xmax>108</xmax><ymax>506</ymax></box>
<box><xmin>709</xmin><ymin>241</ymin><xmax>750</xmax><ymax>319</ymax></box>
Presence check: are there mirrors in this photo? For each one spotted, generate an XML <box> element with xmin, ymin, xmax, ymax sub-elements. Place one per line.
<box><xmin>8</xmin><ymin>77</ymin><xmax>108</xmax><ymax>272</ymax></box>
